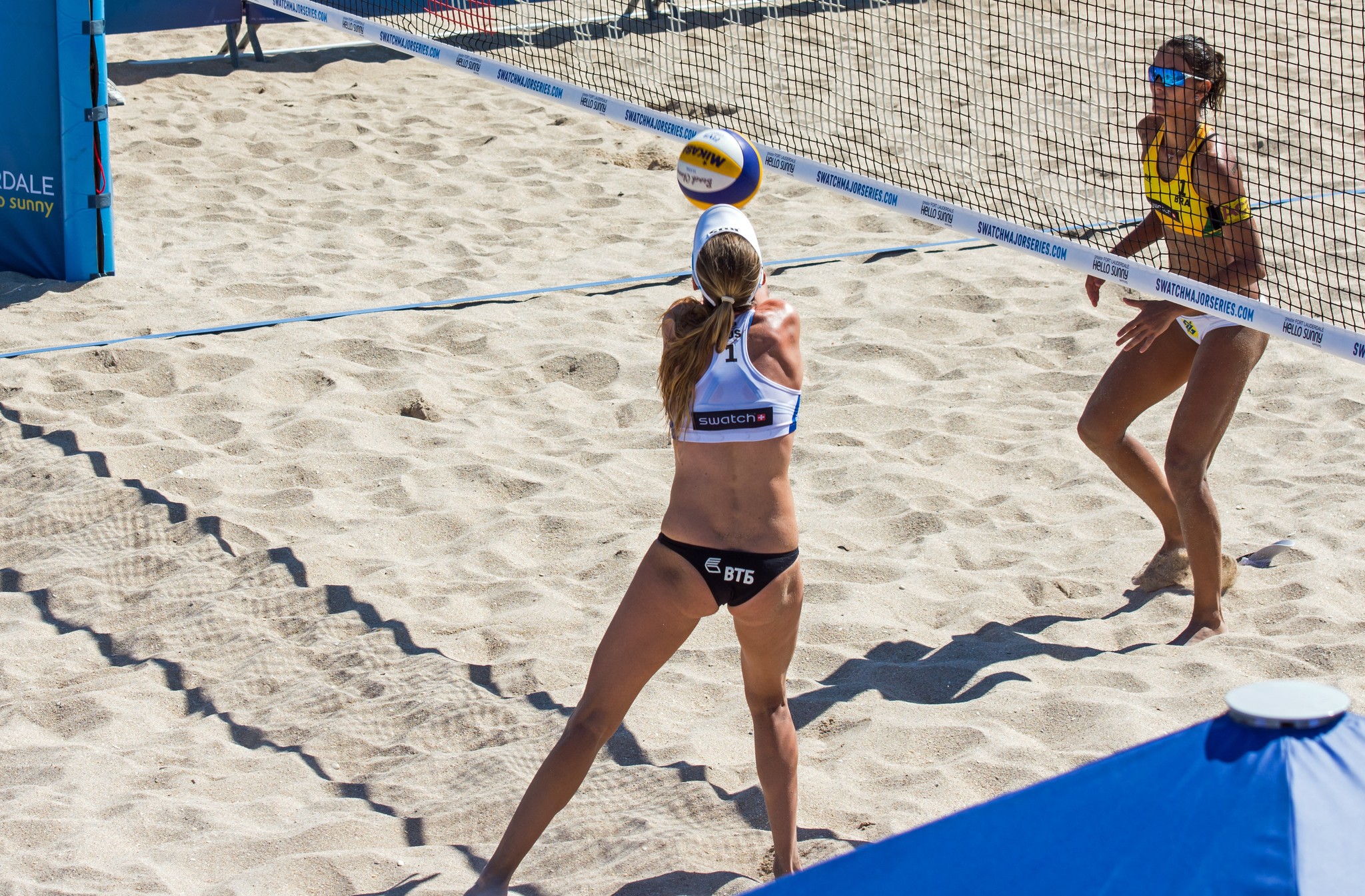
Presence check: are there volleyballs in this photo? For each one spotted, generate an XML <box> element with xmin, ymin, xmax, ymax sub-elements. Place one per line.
<box><xmin>677</xmin><ymin>129</ymin><xmax>763</xmax><ymax>211</ymax></box>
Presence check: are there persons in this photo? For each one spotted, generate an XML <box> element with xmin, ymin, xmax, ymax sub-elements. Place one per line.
<box><xmin>1076</xmin><ymin>34</ymin><xmax>1274</xmax><ymax>648</ymax></box>
<box><xmin>463</xmin><ymin>202</ymin><xmax>815</xmax><ymax>896</ymax></box>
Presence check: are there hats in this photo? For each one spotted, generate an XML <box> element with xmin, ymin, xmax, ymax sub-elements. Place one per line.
<box><xmin>689</xmin><ymin>205</ymin><xmax>766</xmax><ymax>307</ymax></box>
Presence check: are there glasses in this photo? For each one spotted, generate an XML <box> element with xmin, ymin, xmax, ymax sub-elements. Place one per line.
<box><xmin>1148</xmin><ymin>65</ymin><xmax>1205</xmax><ymax>88</ymax></box>
